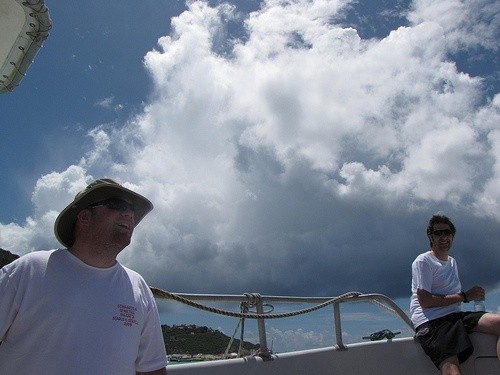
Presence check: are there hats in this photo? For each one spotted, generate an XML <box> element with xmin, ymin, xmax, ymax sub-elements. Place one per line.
<box><xmin>54</xmin><ymin>179</ymin><xmax>153</xmax><ymax>249</ymax></box>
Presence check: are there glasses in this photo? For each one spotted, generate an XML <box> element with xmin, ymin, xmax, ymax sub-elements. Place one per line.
<box><xmin>431</xmin><ymin>229</ymin><xmax>453</xmax><ymax>236</ymax></box>
<box><xmin>84</xmin><ymin>198</ymin><xmax>138</xmax><ymax>212</ymax></box>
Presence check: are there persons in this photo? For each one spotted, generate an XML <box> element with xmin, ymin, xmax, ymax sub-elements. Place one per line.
<box><xmin>409</xmin><ymin>215</ymin><xmax>500</xmax><ymax>375</ymax></box>
<box><xmin>0</xmin><ymin>178</ymin><xmax>170</xmax><ymax>374</ymax></box>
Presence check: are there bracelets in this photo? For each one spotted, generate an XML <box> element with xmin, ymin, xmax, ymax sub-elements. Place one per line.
<box><xmin>461</xmin><ymin>290</ymin><xmax>469</xmax><ymax>302</ymax></box>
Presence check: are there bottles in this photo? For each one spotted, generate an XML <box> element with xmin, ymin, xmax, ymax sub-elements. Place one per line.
<box><xmin>475</xmin><ymin>302</ymin><xmax>485</xmax><ymax>311</ymax></box>
